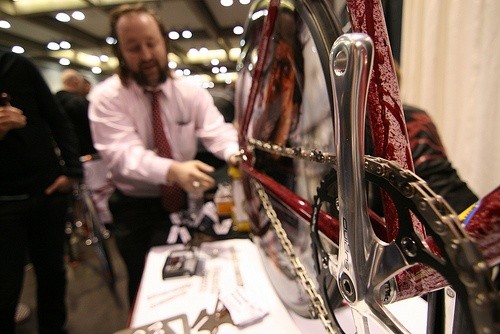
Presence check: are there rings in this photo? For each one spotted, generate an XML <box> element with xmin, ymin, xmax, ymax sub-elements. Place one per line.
<box><xmin>192</xmin><ymin>180</ymin><xmax>200</xmax><ymax>190</ymax></box>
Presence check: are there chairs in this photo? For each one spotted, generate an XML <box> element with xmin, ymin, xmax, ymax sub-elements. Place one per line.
<box><xmin>78</xmin><ymin>151</ymin><xmax>117</xmax><ymax>289</ymax></box>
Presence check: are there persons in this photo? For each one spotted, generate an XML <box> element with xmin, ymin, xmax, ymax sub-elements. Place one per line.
<box><xmin>55</xmin><ymin>67</ymin><xmax>99</xmax><ymax>250</ymax></box>
<box><xmin>0</xmin><ymin>54</ymin><xmax>67</xmax><ymax>334</ymax></box>
<box><xmin>85</xmin><ymin>4</ymin><xmax>243</xmax><ymax>316</ymax></box>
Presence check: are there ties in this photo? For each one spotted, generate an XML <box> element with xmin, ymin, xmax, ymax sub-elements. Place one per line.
<box><xmin>144</xmin><ymin>89</ymin><xmax>187</xmax><ymax>214</ymax></box>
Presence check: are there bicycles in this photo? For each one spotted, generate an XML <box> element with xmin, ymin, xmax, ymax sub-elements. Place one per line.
<box><xmin>68</xmin><ymin>190</ymin><xmax>126</xmax><ymax>311</ymax></box>
<box><xmin>229</xmin><ymin>0</ymin><xmax>500</xmax><ymax>334</ymax></box>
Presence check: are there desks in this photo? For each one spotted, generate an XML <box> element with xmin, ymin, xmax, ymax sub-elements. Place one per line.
<box><xmin>129</xmin><ymin>239</ymin><xmax>454</xmax><ymax>334</ymax></box>
